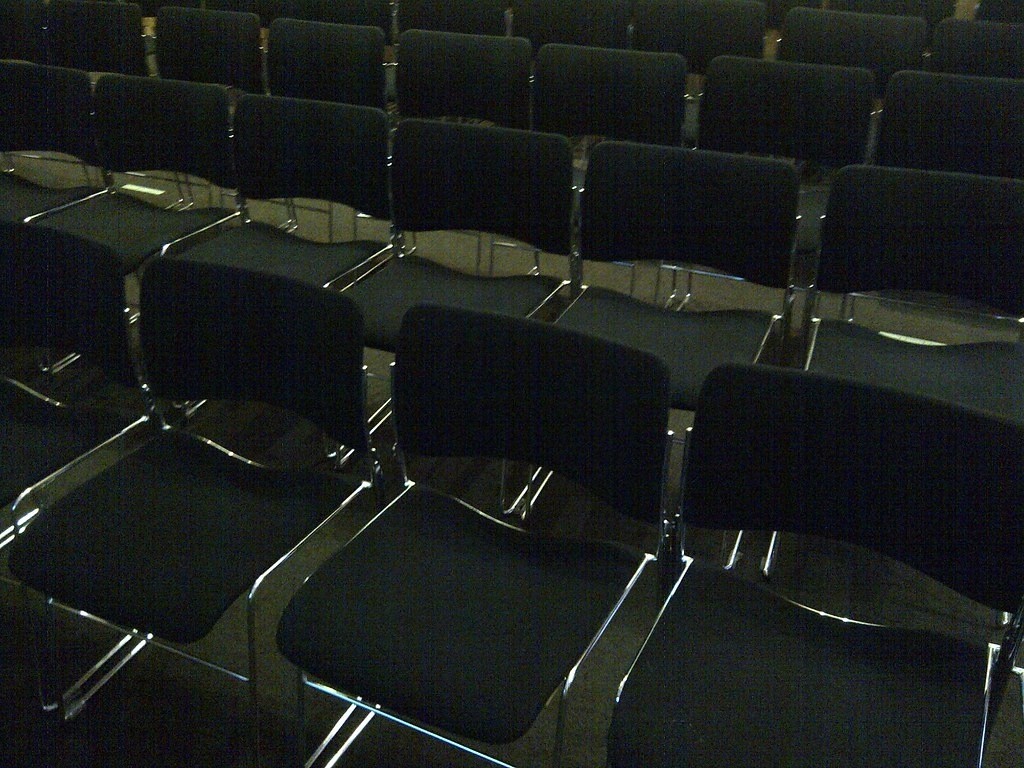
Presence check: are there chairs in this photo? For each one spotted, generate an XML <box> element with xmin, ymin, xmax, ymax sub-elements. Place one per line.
<box><xmin>1</xmin><ymin>1</ymin><xmax>1024</xmax><ymax>768</ymax></box>
